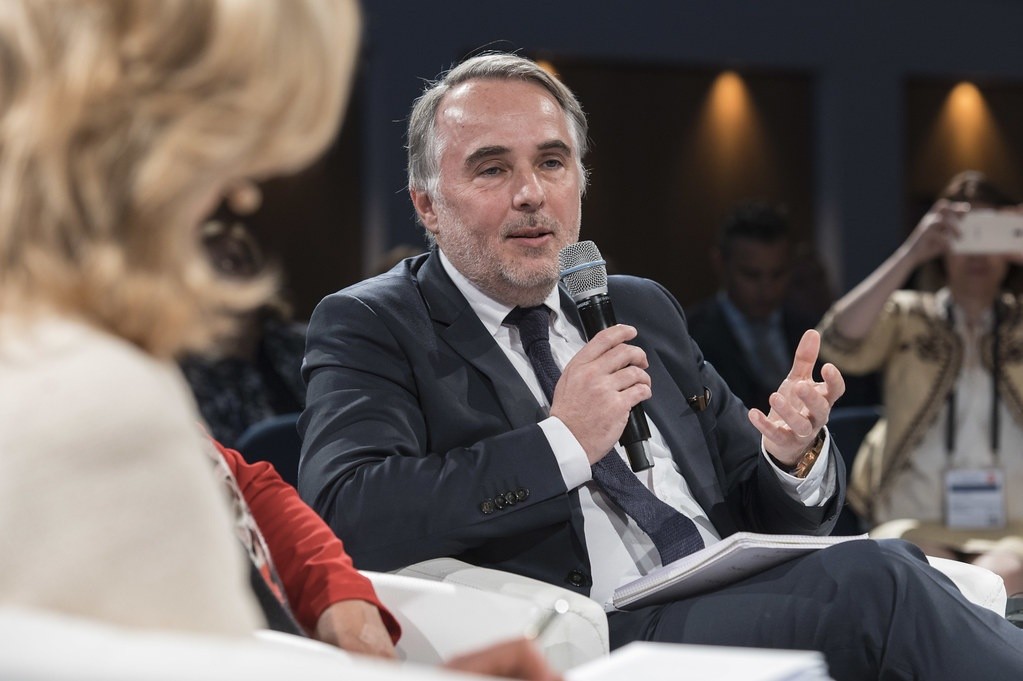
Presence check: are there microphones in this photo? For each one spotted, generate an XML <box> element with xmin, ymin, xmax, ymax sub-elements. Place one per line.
<box><xmin>557</xmin><ymin>242</ymin><xmax>655</xmax><ymax>473</ymax></box>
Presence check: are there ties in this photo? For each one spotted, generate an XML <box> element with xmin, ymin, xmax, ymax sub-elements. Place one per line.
<box><xmin>503</xmin><ymin>307</ymin><xmax>707</xmax><ymax>569</ymax></box>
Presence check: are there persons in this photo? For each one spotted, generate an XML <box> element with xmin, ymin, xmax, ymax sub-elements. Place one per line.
<box><xmin>296</xmin><ymin>57</ymin><xmax>1023</xmax><ymax>680</ymax></box>
<box><xmin>0</xmin><ymin>0</ymin><xmax>565</xmax><ymax>680</ymax></box>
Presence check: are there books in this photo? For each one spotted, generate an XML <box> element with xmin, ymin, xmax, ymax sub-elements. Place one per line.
<box><xmin>612</xmin><ymin>532</ymin><xmax>869</xmax><ymax>609</ymax></box>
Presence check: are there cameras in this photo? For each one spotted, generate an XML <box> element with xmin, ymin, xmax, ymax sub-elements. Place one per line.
<box><xmin>943</xmin><ymin>206</ymin><xmax>1023</xmax><ymax>257</ymax></box>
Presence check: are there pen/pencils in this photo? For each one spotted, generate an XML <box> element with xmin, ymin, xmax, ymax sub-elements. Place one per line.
<box><xmin>534</xmin><ymin>599</ymin><xmax>571</xmax><ymax>642</ymax></box>
<box><xmin>688</xmin><ymin>385</ymin><xmax>712</xmax><ymax>412</ymax></box>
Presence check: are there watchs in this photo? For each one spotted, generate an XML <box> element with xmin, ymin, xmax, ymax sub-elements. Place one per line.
<box><xmin>789</xmin><ymin>435</ymin><xmax>824</xmax><ymax>479</ymax></box>
